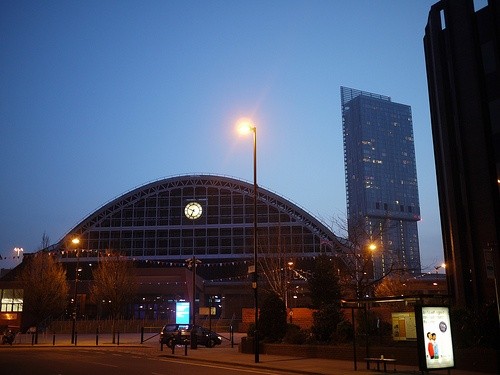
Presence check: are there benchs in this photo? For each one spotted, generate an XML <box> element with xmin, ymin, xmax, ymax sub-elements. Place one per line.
<box><xmin>364</xmin><ymin>358</ymin><xmax>397</xmax><ymax>374</ymax></box>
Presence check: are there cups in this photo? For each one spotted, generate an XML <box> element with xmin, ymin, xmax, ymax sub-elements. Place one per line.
<box><xmin>381</xmin><ymin>355</ymin><xmax>383</xmax><ymax>359</ymax></box>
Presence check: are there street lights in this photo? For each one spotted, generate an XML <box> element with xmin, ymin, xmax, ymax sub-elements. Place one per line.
<box><xmin>237</xmin><ymin>122</ymin><xmax>260</xmax><ymax>363</ymax></box>
<box><xmin>71</xmin><ymin>239</ymin><xmax>80</xmax><ymax>345</ymax></box>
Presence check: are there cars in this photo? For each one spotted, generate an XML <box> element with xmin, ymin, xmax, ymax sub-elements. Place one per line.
<box><xmin>158</xmin><ymin>323</ymin><xmax>222</xmax><ymax>348</ymax></box>
